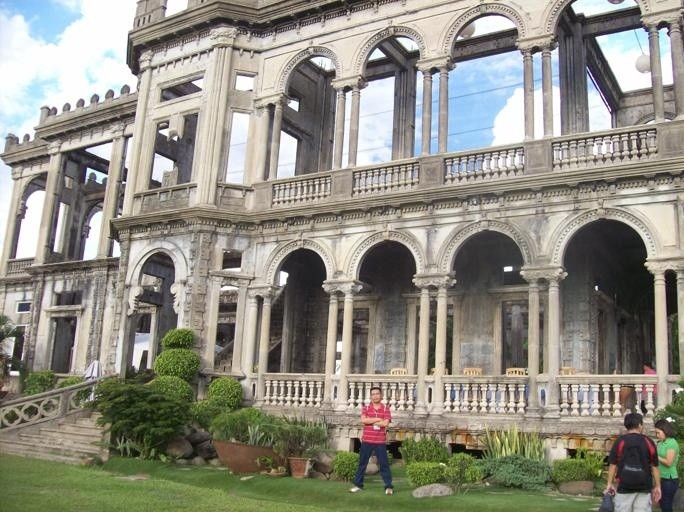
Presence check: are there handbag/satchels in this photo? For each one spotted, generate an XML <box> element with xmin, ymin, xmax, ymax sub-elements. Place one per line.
<box><xmin>600</xmin><ymin>491</ymin><xmax>614</xmax><ymax>512</ymax></box>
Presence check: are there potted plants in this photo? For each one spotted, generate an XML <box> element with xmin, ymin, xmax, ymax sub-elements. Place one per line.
<box><xmin>210</xmin><ymin>430</ymin><xmax>314</xmax><ymax>480</ymax></box>
<box><xmin>553</xmin><ymin>458</ymin><xmax>594</xmax><ymax>498</ymax></box>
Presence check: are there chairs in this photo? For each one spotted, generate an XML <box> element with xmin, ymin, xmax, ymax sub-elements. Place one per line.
<box><xmin>388</xmin><ymin>367</ymin><xmax>579</xmax><ymax>404</ymax></box>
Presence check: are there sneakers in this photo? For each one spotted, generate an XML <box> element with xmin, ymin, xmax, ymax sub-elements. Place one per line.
<box><xmin>348</xmin><ymin>486</ymin><xmax>363</xmax><ymax>493</ymax></box>
<box><xmin>385</xmin><ymin>488</ymin><xmax>394</xmax><ymax>495</ymax></box>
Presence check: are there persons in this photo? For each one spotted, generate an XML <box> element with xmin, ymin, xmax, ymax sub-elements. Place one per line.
<box><xmin>599</xmin><ymin>412</ymin><xmax>663</xmax><ymax>512</ymax></box>
<box><xmin>641</xmin><ymin>362</ymin><xmax>657</xmax><ymax>412</ymax></box>
<box><xmin>349</xmin><ymin>387</ymin><xmax>393</xmax><ymax>495</ymax></box>
<box><xmin>654</xmin><ymin>419</ymin><xmax>680</xmax><ymax>512</ymax></box>
